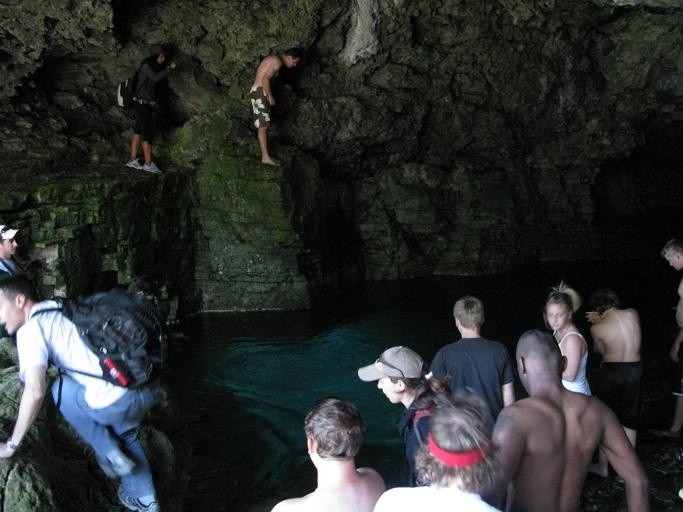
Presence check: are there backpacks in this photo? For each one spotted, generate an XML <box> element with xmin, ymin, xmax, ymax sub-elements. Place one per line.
<box><xmin>118</xmin><ymin>54</ymin><xmax>154</xmax><ymax>108</ymax></box>
<box><xmin>29</xmin><ymin>284</ymin><xmax>158</xmax><ymax>390</ymax></box>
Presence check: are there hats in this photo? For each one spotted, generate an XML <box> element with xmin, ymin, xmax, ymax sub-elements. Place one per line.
<box><xmin>0</xmin><ymin>226</ymin><xmax>19</xmax><ymax>240</ymax></box>
<box><xmin>148</xmin><ymin>44</ymin><xmax>168</xmax><ymax>54</ymax></box>
<box><xmin>358</xmin><ymin>345</ymin><xmax>436</xmax><ymax>389</ymax></box>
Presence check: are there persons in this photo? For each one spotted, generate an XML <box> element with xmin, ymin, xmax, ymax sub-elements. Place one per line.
<box><xmin>0</xmin><ymin>275</ymin><xmax>159</xmax><ymax>512</ymax></box>
<box><xmin>586</xmin><ymin>290</ymin><xmax>644</xmax><ymax>484</ymax></box>
<box><xmin>542</xmin><ymin>283</ymin><xmax>591</xmax><ymax>397</ymax></box>
<box><xmin>118</xmin><ymin>51</ymin><xmax>176</xmax><ymax>175</ymax></box>
<box><xmin>0</xmin><ymin>224</ymin><xmax>21</xmax><ymax>276</ymax></box>
<box><xmin>358</xmin><ymin>345</ymin><xmax>441</xmax><ymax>486</ymax></box>
<box><xmin>270</xmin><ymin>397</ymin><xmax>386</xmax><ymax>512</ymax></box>
<box><xmin>429</xmin><ymin>296</ymin><xmax>515</xmax><ymax>421</ymax></box>
<box><xmin>250</xmin><ymin>48</ymin><xmax>300</xmax><ymax>167</ymax></box>
<box><xmin>491</xmin><ymin>330</ymin><xmax>649</xmax><ymax>511</ymax></box>
<box><xmin>651</xmin><ymin>239</ymin><xmax>682</xmax><ymax>441</ymax></box>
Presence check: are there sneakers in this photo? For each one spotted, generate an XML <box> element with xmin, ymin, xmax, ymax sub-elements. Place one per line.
<box><xmin>99</xmin><ymin>456</ymin><xmax>138</xmax><ymax>480</ymax></box>
<box><xmin>142</xmin><ymin>161</ymin><xmax>163</xmax><ymax>174</ymax></box>
<box><xmin>117</xmin><ymin>487</ymin><xmax>161</xmax><ymax>511</ymax></box>
<box><xmin>125</xmin><ymin>158</ymin><xmax>142</xmax><ymax>170</ymax></box>
<box><xmin>647</xmin><ymin>427</ymin><xmax>680</xmax><ymax>441</ymax></box>
